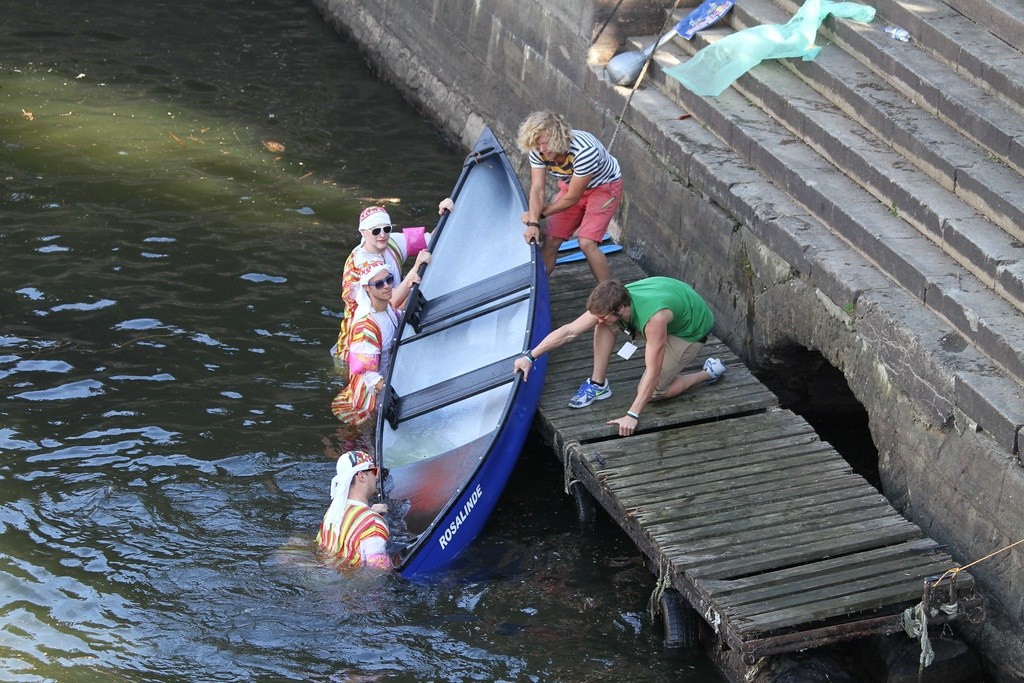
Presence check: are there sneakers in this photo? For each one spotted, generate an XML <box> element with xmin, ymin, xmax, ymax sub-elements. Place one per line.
<box><xmin>702</xmin><ymin>357</ymin><xmax>728</xmax><ymax>384</ymax></box>
<box><xmin>568</xmin><ymin>377</ymin><xmax>613</xmax><ymax>409</ymax></box>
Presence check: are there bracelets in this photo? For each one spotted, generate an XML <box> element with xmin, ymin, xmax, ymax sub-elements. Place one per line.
<box><xmin>541</xmin><ymin>211</ymin><xmax>546</xmax><ymax>220</ymax></box>
<box><xmin>626</xmin><ymin>411</ymin><xmax>640</xmax><ymax>423</ymax></box>
<box><xmin>527</xmin><ymin>222</ymin><xmax>540</xmax><ymax>229</ymax></box>
<box><xmin>525</xmin><ymin>350</ymin><xmax>537</xmax><ymax>364</ymax></box>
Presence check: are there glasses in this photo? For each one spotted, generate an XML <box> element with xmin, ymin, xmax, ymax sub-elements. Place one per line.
<box><xmin>598</xmin><ymin>310</ymin><xmax>615</xmax><ymax>321</ymax></box>
<box><xmin>367</xmin><ymin>225</ymin><xmax>392</xmax><ymax>237</ymax></box>
<box><xmin>361</xmin><ymin>467</ymin><xmax>379</xmax><ymax>476</ymax></box>
<box><xmin>367</xmin><ymin>272</ymin><xmax>395</xmax><ymax>290</ymax></box>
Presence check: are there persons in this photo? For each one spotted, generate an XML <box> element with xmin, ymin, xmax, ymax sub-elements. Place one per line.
<box><xmin>518</xmin><ymin>110</ymin><xmax>622</xmax><ymax>284</ymax></box>
<box><xmin>338</xmin><ymin>197</ymin><xmax>455</xmax><ymax>350</ymax></box>
<box><xmin>515</xmin><ymin>277</ymin><xmax>727</xmax><ymax>437</ymax></box>
<box><xmin>348</xmin><ymin>257</ymin><xmax>405</xmax><ymax>409</ymax></box>
<box><xmin>319</xmin><ymin>450</ymin><xmax>390</xmax><ymax>569</ymax></box>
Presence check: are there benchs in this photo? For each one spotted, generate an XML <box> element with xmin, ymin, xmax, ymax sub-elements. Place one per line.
<box><xmin>380</xmin><ymin>260</ymin><xmax>533</xmax><ymax>430</ymax></box>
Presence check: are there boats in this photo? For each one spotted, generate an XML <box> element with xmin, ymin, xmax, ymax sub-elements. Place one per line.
<box><xmin>376</xmin><ymin>124</ymin><xmax>551</xmax><ymax>584</ymax></box>
